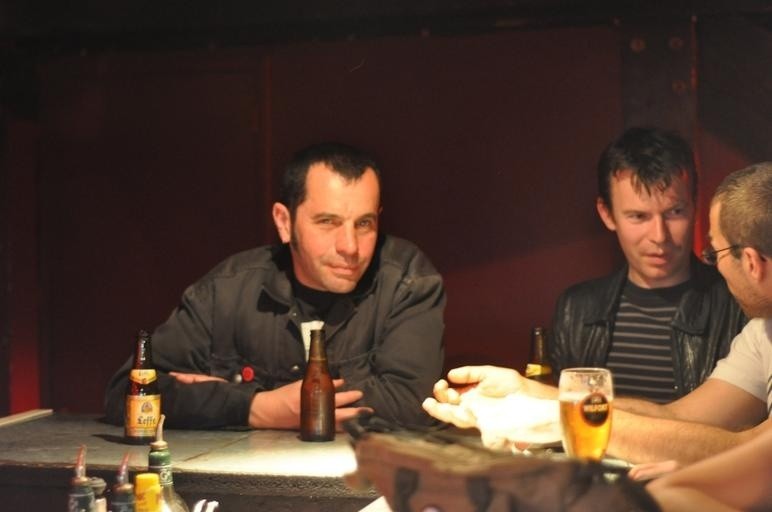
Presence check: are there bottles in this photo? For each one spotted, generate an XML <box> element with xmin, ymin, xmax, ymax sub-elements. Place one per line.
<box><xmin>123</xmin><ymin>330</ymin><xmax>161</xmax><ymax>445</ymax></box>
<box><xmin>134</xmin><ymin>473</ymin><xmax>171</xmax><ymax>512</ymax></box>
<box><xmin>109</xmin><ymin>448</ymin><xmax>135</xmax><ymax>512</ymax></box>
<box><xmin>67</xmin><ymin>445</ymin><xmax>97</xmax><ymax>512</ymax></box>
<box><xmin>300</xmin><ymin>329</ymin><xmax>334</xmax><ymax>441</ymax></box>
<box><xmin>89</xmin><ymin>477</ymin><xmax>107</xmax><ymax>512</ymax></box>
<box><xmin>524</xmin><ymin>325</ymin><xmax>555</xmax><ymax>386</ymax></box>
<box><xmin>148</xmin><ymin>414</ymin><xmax>190</xmax><ymax>512</ymax></box>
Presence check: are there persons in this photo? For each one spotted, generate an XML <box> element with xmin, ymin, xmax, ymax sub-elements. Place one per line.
<box><xmin>543</xmin><ymin>125</ymin><xmax>748</xmax><ymax>483</ymax></box>
<box><xmin>103</xmin><ymin>141</ymin><xmax>446</xmax><ymax>450</ymax></box>
<box><xmin>422</xmin><ymin>162</ymin><xmax>772</xmax><ymax>466</ymax></box>
<box><xmin>642</xmin><ymin>427</ymin><xmax>772</xmax><ymax>512</ymax></box>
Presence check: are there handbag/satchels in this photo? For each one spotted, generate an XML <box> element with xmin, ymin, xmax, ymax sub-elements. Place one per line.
<box><xmin>350</xmin><ymin>412</ymin><xmax>664</xmax><ymax>511</ymax></box>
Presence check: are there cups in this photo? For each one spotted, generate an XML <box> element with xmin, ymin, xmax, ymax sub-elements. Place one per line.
<box><xmin>559</xmin><ymin>367</ymin><xmax>614</xmax><ymax>462</ymax></box>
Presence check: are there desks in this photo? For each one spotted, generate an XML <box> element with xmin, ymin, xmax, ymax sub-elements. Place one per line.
<box><xmin>0</xmin><ymin>404</ymin><xmax>382</xmax><ymax>512</ymax></box>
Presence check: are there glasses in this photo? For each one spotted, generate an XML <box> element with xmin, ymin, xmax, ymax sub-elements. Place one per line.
<box><xmin>700</xmin><ymin>243</ymin><xmax>741</xmax><ymax>265</ymax></box>
